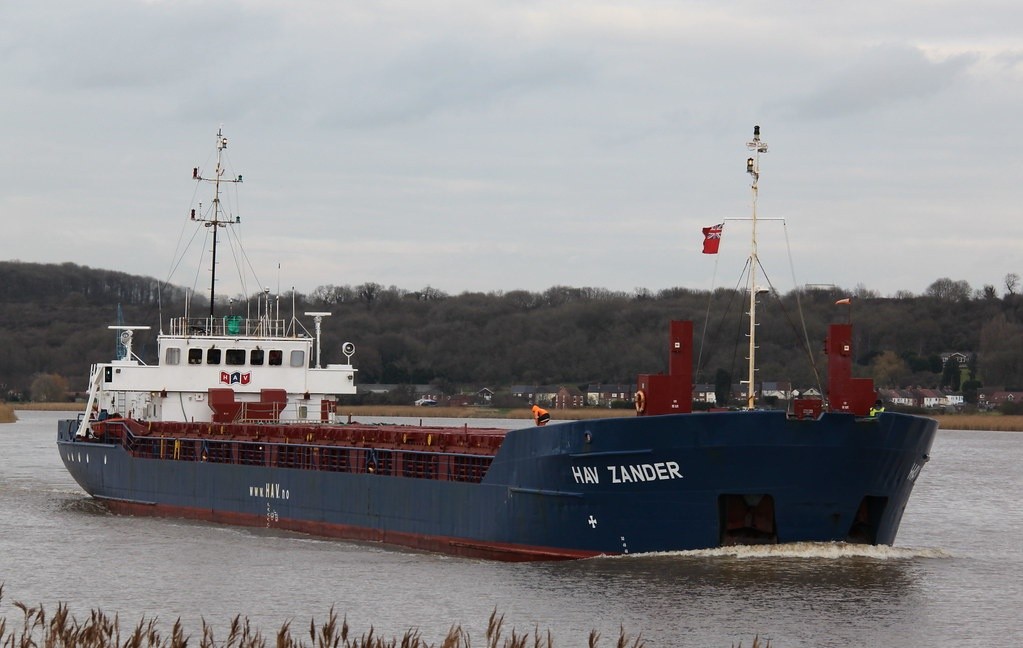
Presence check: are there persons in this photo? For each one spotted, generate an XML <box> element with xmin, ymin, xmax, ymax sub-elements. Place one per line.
<box><xmin>532</xmin><ymin>405</ymin><xmax>550</xmax><ymax>426</ymax></box>
<box><xmin>869</xmin><ymin>400</ymin><xmax>885</xmax><ymax>416</ymax></box>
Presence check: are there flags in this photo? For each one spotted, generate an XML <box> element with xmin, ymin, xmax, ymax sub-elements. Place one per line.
<box><xmin>702</xmin><ymin>224</ymin><xmax>723</xmax><ymax>255</ymax></box>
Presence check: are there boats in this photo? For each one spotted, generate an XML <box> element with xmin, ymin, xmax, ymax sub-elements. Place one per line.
<box><xmin>56</xmin><ymin>123</ymin><xmax>940</xmax><ymax>561</ymax></box>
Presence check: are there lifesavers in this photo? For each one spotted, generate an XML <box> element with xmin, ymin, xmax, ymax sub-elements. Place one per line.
<box><xmin>634</xmin><ymin>391</ymin><xmax>645</xmax><ymax>413</ymax></box>
